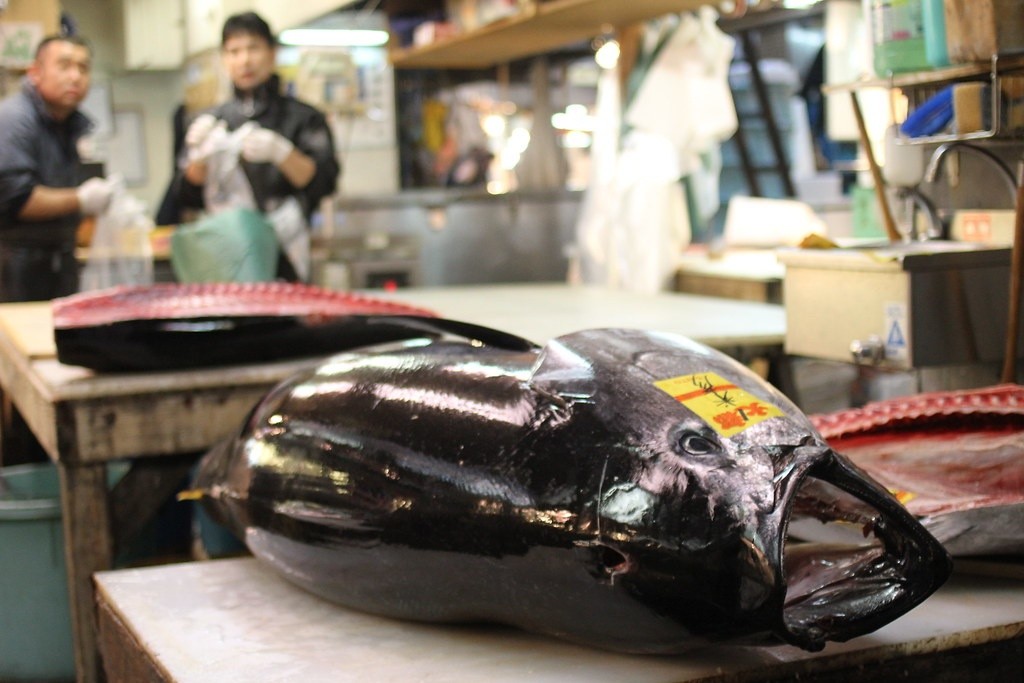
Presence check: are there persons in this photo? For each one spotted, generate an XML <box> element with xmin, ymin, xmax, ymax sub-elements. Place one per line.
<box><xmin>153</xmin><ymin>11</ymin><xmax>340</xmax><ymax>288</ymax></box>
<box><xmin>0</xmin><ymin>33</ymin><xmax>124</xmax><ymax>470</ymax></box>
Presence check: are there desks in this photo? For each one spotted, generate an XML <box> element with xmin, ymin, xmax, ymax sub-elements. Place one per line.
<box><xmin>0</xmin><ymin>283</ymin><xmax>1024</xmax><ymax>683</ymax></box>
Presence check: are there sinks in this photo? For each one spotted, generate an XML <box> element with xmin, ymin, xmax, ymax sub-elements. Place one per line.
<box><xmin>775</xmin><ymin>236</ymin><xmax>1024</xmax><ymax>370</ymax></box>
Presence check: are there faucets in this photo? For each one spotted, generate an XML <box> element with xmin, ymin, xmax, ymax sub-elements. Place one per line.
<box><xmin>924</xmin><ymin>139</ymin><xmax>1020</xmax><ymax>212</ymax></box>
<box><xmin>892</xmin><ymin>183</ymin><xmax>946</xmax><ymax>240</ymax></box>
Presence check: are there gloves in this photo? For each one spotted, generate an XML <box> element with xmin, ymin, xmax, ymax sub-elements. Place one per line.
<box><xmin>236</xmin><ymin>121</ymin><xmax>292</xmax><ymax>165</ymax></box>
<box><xmin>76</xmin><ymin>175</ymin><xmax>111</xmax><ymax>215</ymax></box>
<box><xmin>182</xmin><ymin>114</ymin><xmax>234</xmax><ymax>164</ymax></box>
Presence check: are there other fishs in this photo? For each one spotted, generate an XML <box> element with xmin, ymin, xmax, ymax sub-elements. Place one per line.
<box><xmin>53</xmin><ymin>261</ymin><xmax>1023</xmax><ymax>650</ymax></box>
